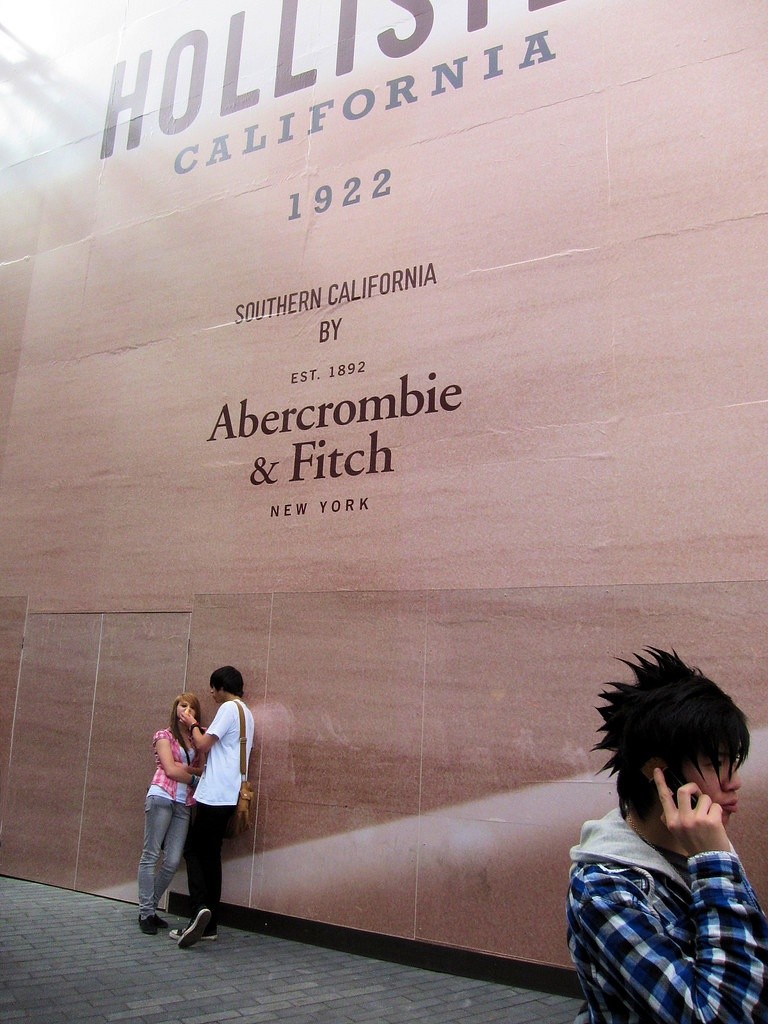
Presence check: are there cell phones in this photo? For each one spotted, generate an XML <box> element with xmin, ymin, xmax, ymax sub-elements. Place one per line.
<box><xmin>642</xmin><ymin>756</ymin><xmax>698</xmax><ymax>812</ymax></box>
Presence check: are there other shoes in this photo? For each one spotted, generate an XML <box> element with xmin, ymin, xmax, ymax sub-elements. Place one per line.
<box><xmin>139</xmin><ymin>915</ymin><xmax>157</xmax><ymax>934</ymax></box>
<box><xmin>139</xmin><ymin>913</ymin><xmax>169</xmax><ymax>928</ymax></box>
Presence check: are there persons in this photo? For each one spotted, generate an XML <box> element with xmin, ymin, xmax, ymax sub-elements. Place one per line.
<box><xmin>168</xmin><ymin>665</ymin><xmax>254</xmax><ymax>948</ymax></box>
<box><xmin>566</xmin><ymin>645</ymin><xmax>768</xmax><ymax>1024</ymax></box>
<box><xmin>138</xmin><ymin>693</ymin><xmax>204</xmax><ymax>935</ymax></box>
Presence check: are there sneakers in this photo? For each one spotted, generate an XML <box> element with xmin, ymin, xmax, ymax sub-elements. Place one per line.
<box><xmin>169</xmin><ymin>921</ymin><xmax>218</xmax><ymax>941</ymax></box>
<box><xmin>177</xmin><ymin>904</ymin><xmax>211</xmax><ymax>948</ymax></box>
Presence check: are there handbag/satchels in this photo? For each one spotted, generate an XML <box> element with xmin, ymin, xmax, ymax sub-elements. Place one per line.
<box><xmin>225</xmin><ymin>782</ymin><xmax>253</xmax><ymax>839</ymax></box>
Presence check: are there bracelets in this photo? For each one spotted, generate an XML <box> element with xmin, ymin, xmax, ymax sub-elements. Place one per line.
<box><xmin>190</xmin><ymin>723</ymin><xmax>203</xmax><ymax>736</ymax></box>
